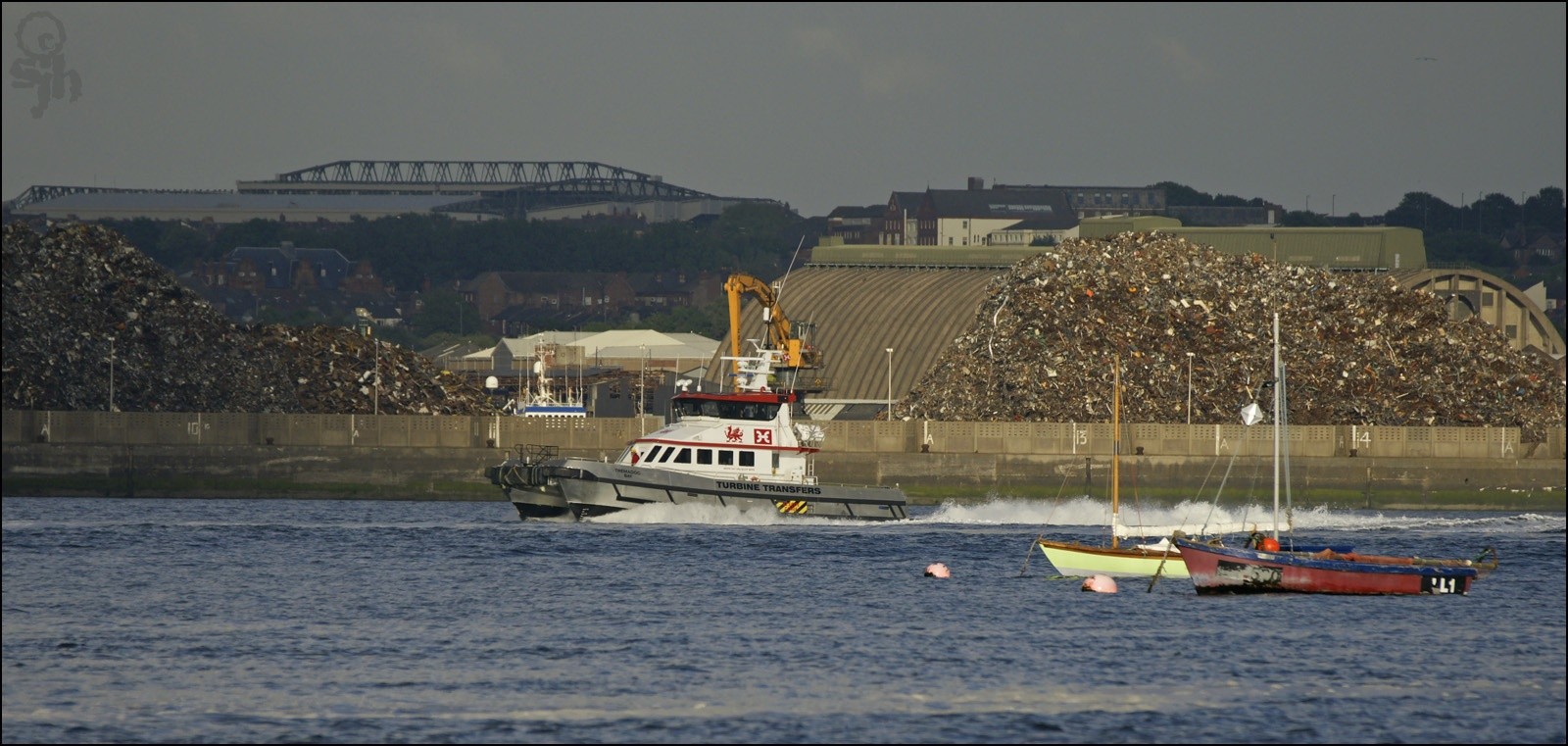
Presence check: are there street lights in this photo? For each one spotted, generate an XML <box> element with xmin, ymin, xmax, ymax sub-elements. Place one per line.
<box><xmin>375</xmin><ymin>338</ymin><xmax>379</xmax><ymax>417</ymax></box>
<box><xmin>107</xmin><ymin>337</ymin><xmax>115</xmax><ymax>414</ymax></box>
<box><xmin>886</xmin><ymin>348</ymin><xmax>893</xmax><ymax>422</ymax></box>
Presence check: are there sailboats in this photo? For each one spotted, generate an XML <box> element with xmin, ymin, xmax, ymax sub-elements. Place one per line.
<box><xmin>1038</xmin><ymin>354</ymin><xmax>1192</xmax><ymax>578</ymax></box>
<box><xmin>1166</xmin><ymin>232</ymin><xmax>1497</xmax><ymax>597</ymax></box>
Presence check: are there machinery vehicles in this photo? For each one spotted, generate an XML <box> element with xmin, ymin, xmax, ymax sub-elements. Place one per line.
<box><xmin>724</xmin><ymin>274</ymin><xmax>826</xmax><ymax>394</ymax></box>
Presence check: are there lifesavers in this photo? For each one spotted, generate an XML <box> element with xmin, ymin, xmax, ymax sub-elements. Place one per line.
<box><xmin>760</xmin><ymin>386</ymin><xmax>766</xmax><ymax>393</ymax></box>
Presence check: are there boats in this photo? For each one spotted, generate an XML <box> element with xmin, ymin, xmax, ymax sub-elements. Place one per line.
<box><xmin>484</xmin><ymin>233</ymin><xmax>915</xmax><ymax>520</ymax></box>
